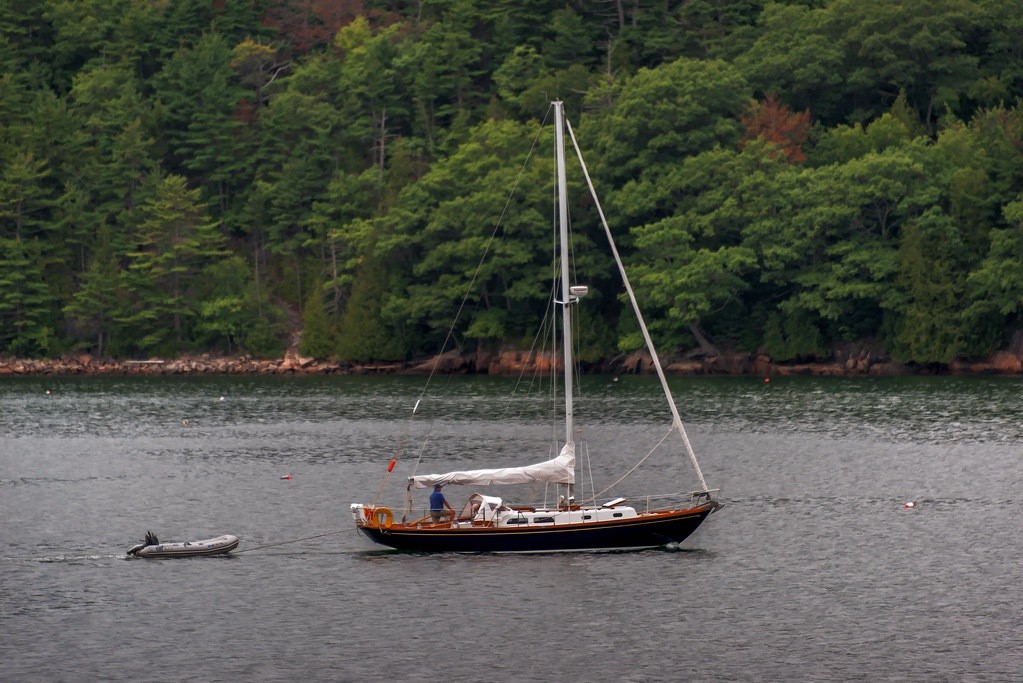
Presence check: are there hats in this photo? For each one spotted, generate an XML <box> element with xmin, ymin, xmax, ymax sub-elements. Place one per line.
<box><xmin>434</xmin><ymin>484</ymin><xmax>442</xmax><ymax>488</ymax></box>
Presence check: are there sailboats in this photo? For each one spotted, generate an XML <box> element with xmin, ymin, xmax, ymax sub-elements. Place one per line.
<box><xmin>348</xmin><ymin>99</ymin><xmax>729</xmax><ymax>560</ymax></box>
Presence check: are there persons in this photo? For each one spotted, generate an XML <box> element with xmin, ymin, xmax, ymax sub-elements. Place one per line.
<box><xmin>429</xmin><ymin>484</ymin><xmax>455</xmax><ymax>524</ymax></box>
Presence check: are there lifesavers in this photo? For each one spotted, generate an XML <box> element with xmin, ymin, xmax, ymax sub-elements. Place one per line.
<box><xmin>373</xmin><ymin>507</ymin><xmax>394</xmax><ymax>528</ymax></box>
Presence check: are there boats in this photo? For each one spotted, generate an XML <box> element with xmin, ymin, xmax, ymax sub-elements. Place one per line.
<box><xmin>127</xmin><ymin>534</ymin><xmax>241</xmax><ymax>560</ymax></box>
